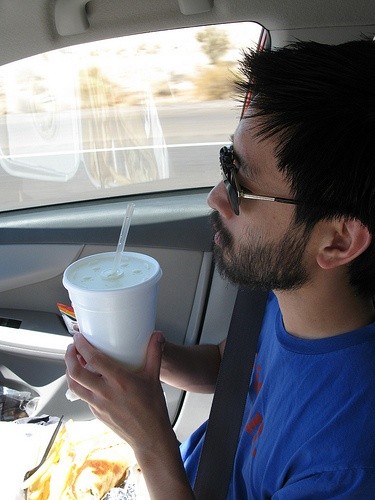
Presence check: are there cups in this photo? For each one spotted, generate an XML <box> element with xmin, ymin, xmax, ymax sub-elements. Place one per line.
<box><xmin>62</xmin><ymin>251</ymin><xmax>162</xmax><ymax>372</ymax></box>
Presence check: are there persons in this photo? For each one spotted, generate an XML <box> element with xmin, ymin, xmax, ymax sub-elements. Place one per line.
<box><xmin>64</xmin><ymin>39</ymin><xmax>375</xmax><ymax>500</ymax></box>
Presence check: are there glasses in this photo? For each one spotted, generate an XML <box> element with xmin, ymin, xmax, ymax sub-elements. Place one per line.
<box><xmin>220</xmin><ymin>145</ymin><xmax>306</xmax><ymax>215</ymax></box>
